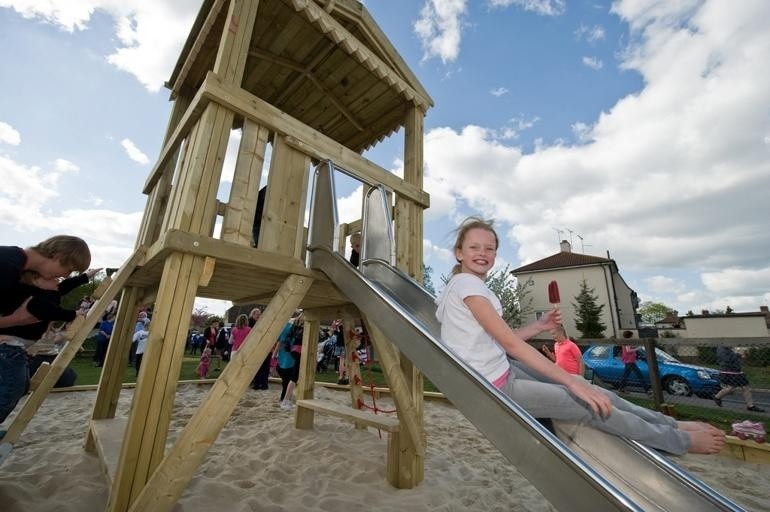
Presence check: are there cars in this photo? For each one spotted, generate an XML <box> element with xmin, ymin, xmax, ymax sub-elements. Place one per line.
<box><xmin>581</xmin><ymin>343</ymin><xmax>721</xmax><ymax>400</ymax></box>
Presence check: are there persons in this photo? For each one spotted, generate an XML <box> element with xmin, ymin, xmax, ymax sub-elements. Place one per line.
<box><xmin>619</xmin><ymin>330</ymin><xmax>655</xmax><ymax>398</ymax></box>
<box><xmin>185</xmin><ymin>307</ymin><xmax>370</xmax><ymax>410</ymax></box>
<box><xmin>0</xmin><ymin>235</ymin><xmax>157</xmax><ymax>442</ymax></box>
<box><xmin>435</xmin><ymin>214</ymin><xmax>729</xmax><ymax>454</ymax></box>
<box><xmin>348</xmin><ymin>232</ymin><xmax>361</xmax><ymax>268</ymax></box>
<box><xmin>542</xmin><ymin>323</ymin><xmax>585</xmax><ymax>378</ymax></box>
<box><xmin>252</xmin><ymin>185</ymin><xmax>267</xmax><ymax>247</ymax></box>
<box><xmin>712</xmin><ymin>343</ymin><xmax>765</xmax><ymax>412</ymax></box>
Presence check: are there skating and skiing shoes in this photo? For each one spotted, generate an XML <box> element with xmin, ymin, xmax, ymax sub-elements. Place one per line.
<box><xmin>731</xmin><ymin>421</ymin><xmax>767</xmax><ymax>443</ymax></box>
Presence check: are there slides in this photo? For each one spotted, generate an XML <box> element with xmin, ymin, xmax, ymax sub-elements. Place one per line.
<box><xmin>306</xmin><ymin>246</ymin><xmax>743</xmax><ymax>512</ymax></box>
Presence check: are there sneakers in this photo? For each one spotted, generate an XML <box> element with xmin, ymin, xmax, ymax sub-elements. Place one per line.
<box><xmin>280</xmin><ymin>400</ymin><xmax>296</xmax><ymax>409</ymax></box>
<box><xmin>338</xmin><ymin>378</ymin><xmax>349</xmax><ymax>385</ymax></box>
<box><xmin>713</xmin><ymin>395</ymin><xmax>722</xmax><ymax>407</ymax></box>
<box><xmin>747</xmin><ymin>406</ymin><xmax>764</xmax><ymax>412</ymax></box>
<box><xmin>253</xmin><ymin>385</ymin><xmax>268</xmax><ymax>390</ymax></box>
<box><xmin>618</xmin><ymin>388</ymin><xmax>629</xmax><ymax>393</ymax></box>
<box><xmin>647</xmin><ymin>389</ymin><xmax>654</xmax><ymax>398</ymax></box>
